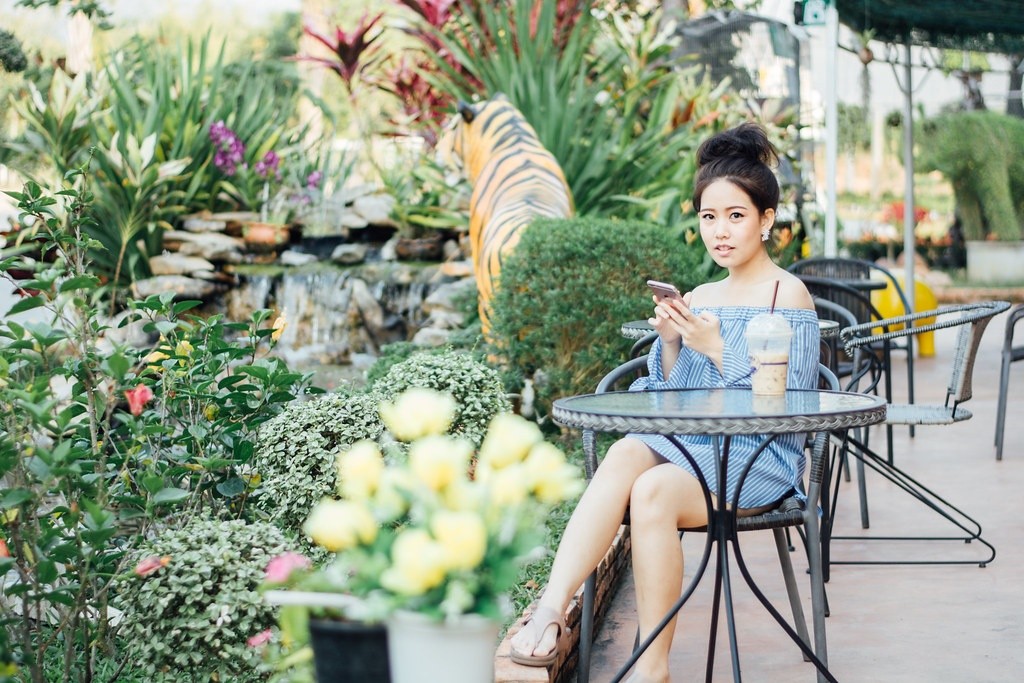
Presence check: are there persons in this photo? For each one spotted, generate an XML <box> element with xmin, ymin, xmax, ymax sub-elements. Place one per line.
<box><xmin>507</xmin><ymin>119</ymin><xmax>823</xmax><ymax>683</ymax></box>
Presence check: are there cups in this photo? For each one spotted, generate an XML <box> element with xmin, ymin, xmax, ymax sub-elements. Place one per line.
<box><xmin>743</xmin><ymin>312</ymin><xmax>793</xmax><ymax>398</ymax></box>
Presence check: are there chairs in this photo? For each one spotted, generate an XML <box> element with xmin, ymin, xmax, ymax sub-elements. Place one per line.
<box><xmin>575</xmin><ymin>258</ymin><xmax>1011</xmax><ymax>683</ymax></box>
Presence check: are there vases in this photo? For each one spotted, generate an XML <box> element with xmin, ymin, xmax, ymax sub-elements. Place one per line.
<box><xmin>243</xmin><ymin>221</ymin><xmax>290</xmax><ymax>254</ymax></box>
<box><xmin>385</xmin><ymin>603</ymin><xmax>500</xmax><ymax>683</ymax></box>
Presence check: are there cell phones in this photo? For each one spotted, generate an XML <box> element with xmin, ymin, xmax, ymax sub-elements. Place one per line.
<box><xmin>647</xmin><ymin>280</ymin><xmax>688</xmax><ymax>310</ymax></box>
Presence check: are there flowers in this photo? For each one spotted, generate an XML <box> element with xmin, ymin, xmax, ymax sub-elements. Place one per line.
<box><xmin>304</xmin><ymin>389</ymin><xmax>583</xmax><ymax>630</ymax></box>
<box><xmin>210</xmin><ymin>119</ymin><xmax>321</xmax><ymax>222</ymax></box>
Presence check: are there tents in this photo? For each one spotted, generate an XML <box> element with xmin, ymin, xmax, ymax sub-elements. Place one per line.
<box><xmin>791</xmin><ymin>0</ymin><xmax>1023</xmax><ymax>357</ymax></box>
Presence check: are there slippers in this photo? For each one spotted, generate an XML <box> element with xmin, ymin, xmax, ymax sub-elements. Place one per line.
<box><xmin>512</xmin><ymin>609</ymin><xmax>571</xmax><ymax>664</ymax></box>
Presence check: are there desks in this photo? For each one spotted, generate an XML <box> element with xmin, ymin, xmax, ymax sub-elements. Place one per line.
<box><xmin>620</xmin><ymin>318</ymin><xmax>838</xmax><ymax>616</ymax></box>
<box><xmin>794</xmin><ymin>277</ymin><xmax>888</xmax><ymax>377</ymax></box>
<box><xmin>550</xmin><ymin>387</ymin><xmax>888</xmax><ymax>683</ymax></box>
